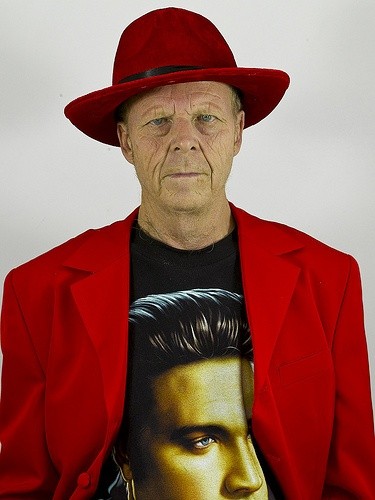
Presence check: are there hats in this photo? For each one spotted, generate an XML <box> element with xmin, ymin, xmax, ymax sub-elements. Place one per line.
<box><xmin>64</xmin><ymin>7</ymin><xmax>290</xmax><ymax>147</ymax></box>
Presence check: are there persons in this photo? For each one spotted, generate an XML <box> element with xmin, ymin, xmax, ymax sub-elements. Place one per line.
<box><xmin>0</xmin><ymin>6</ymin><xmax>375</xmax><ymax>500</ymax></box>
